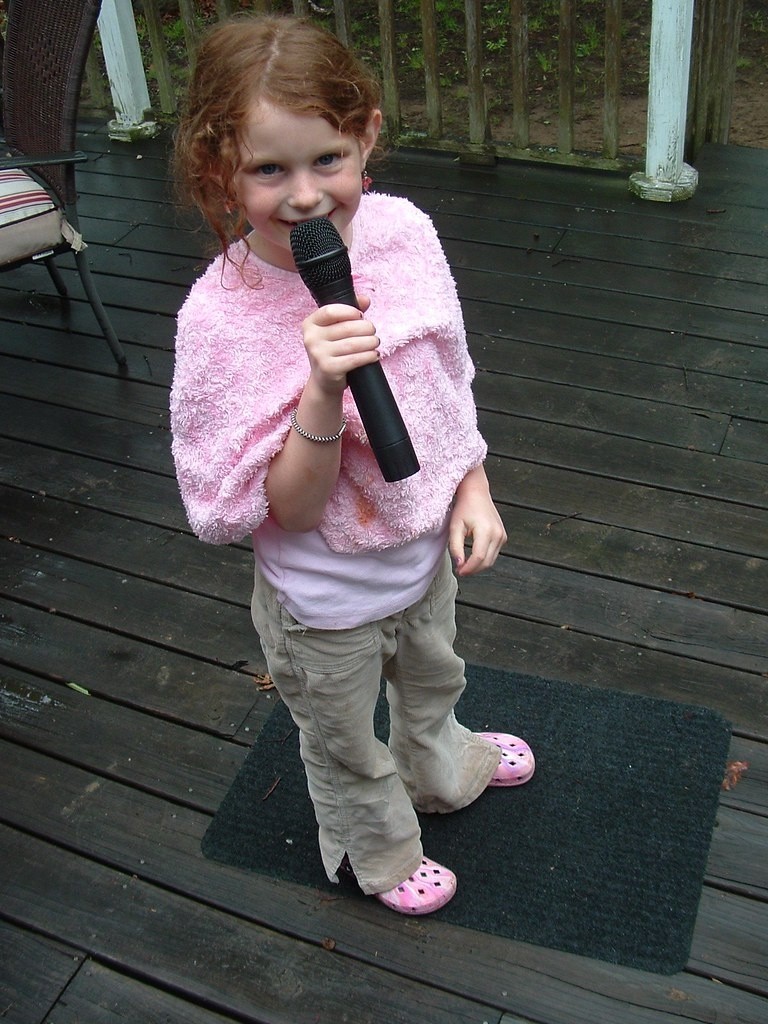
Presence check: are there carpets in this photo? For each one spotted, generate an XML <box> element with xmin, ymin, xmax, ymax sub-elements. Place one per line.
<box><xmin>200</xmin><ymin>659</ymin><xmax>733</xmax><ymax>976</ymax></box>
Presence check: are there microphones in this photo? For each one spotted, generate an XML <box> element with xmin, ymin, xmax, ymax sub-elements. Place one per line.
<box><xmin>290</xmin><ymin>218</ymin><xmax>421</xmax><ymax>484</ymax></box>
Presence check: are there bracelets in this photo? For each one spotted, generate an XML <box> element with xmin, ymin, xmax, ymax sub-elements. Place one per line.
<box><xmin>291</xmin><ymin>407</ymin><xmax>347</xmax><ymax>443</ymax></box>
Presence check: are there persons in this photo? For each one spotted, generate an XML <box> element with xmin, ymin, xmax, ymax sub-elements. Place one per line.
<box><xmin>169</xmin><ymin>11</ymin><xmax>538</xmax><ymax>916</ymax></box>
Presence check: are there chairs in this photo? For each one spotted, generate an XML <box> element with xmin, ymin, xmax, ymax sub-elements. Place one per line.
<box><xmin>0</xmin><ymin>0</ymin><xmax>128</xmax><ymax>368</ymax></box>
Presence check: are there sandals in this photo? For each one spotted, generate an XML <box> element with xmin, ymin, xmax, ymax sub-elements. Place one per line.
<box><xmin>340</xmin><ymin>855</ymin><xmax>457</xmax><ymax>914</ymax></box>
<box><xmin>473</xmin><ymin>732</ymin><xmax>535</xmax><ymax>786</ymax></box>
